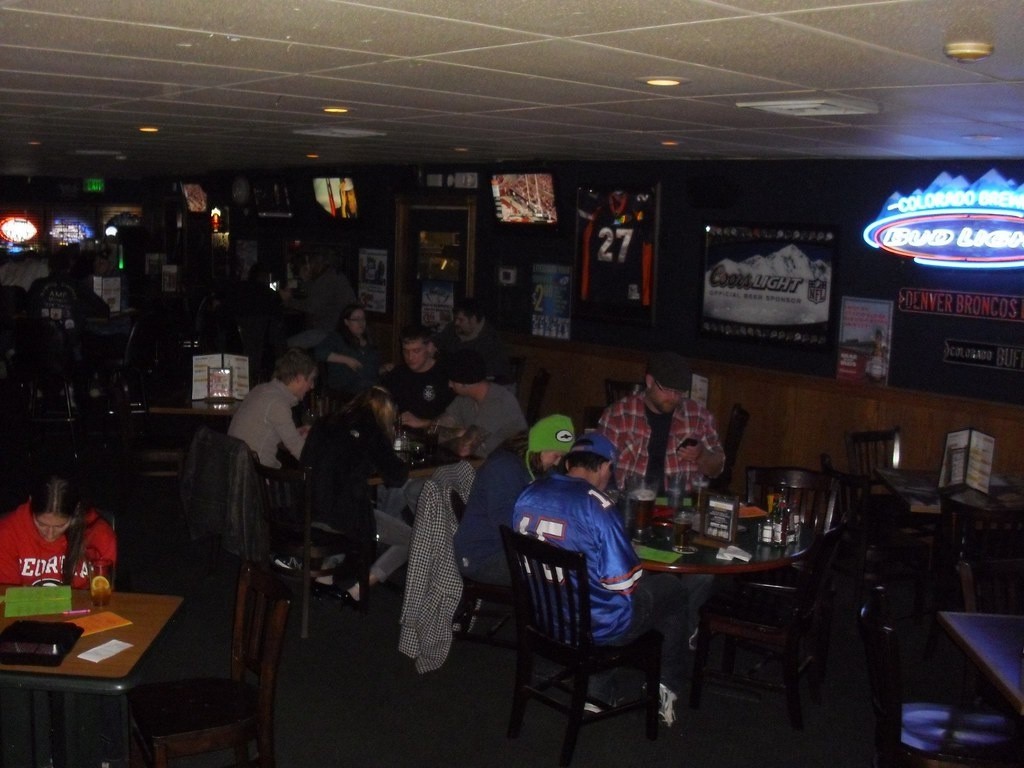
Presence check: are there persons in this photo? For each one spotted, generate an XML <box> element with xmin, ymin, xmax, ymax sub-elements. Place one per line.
<box><xmin>85</xmin><ymin>250</ymin><xmax>130</xmax><ymax>353</ymax></box>
<box><xmin>228</xmin><ymin>253</ymin><xmax>356</xmax><ymax>355</ymax></box>
<box><xmin>227</xmin><ymin>347</ymin><xmax>320</xmax><ymax>568</ymax></box>
<box><xmin>450</xmin><ymin>297</ymin><xmax>510</xmax><ymax>381</ymax></box>
<box><xmin>0</xmin><ymin>461</ymin><xmax>117</xmax><ymax>590</ymax></box>
<box><xmin>300</xmin><ymin>386</ymin><xmax>414</xmax><ymax>613</ymax></box>
<box><xmin>315</xmin><ymin>306</ymin><xmax>395</xmax><ymax>396</ymax></box>
<box><xmin>454</xmin><ymin>414</ymin><xmax>575</xmax><ymax>588</ymax></box>
<box><xmin>403</xmin><ymin>349</ymin><xmax>528</xmax><ymax>516</ymax></box>
<box><xmin>514</xmin><ymin>432</ymin><xmax>691</xmax><ymax>727</ymax></box>
<box><xmin>597</xmin><ymin>352</ymin><xmax>726</xmax><ymax>652</ymax></box>
<box><xmin>374</xmin><ymin>324</ymin><xmax>468</xmax><ymax>521</ymax></box>
<box><xmin>26</xmin><ymin>256</ymin><xmax>110</xmax><ymax>353</ymax></box>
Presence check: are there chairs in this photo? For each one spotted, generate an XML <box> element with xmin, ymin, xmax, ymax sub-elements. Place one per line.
<box><xmin>0</xmin><ymin>283</ymin><xmax>1024</xmax><ymax>768</ymax></box>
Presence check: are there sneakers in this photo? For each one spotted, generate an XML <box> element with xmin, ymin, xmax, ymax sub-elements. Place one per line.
<box><xmin>583</xmin><ymin>697</ymin><xmax>616</xmax><ymax>715</ymax></box>
<box><xmin>643</xmin><ymin>682</ymin><xmax>677</xmax><ymax>729</ymax></box>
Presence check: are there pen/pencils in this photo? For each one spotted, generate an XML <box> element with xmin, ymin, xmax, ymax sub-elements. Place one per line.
<box><xmin>62</xmin><ymin>609</ymin><xmax>91</xmax><ymax>614</ymax></box>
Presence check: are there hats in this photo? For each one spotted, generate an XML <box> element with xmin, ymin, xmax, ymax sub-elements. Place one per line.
<box><xmin>569</xmin><ymin>433</ymin><xmax>617</xmax><ymax>472</ymax></box>
<box><xmin>648</xmin><ymin>351</ymin><xmax>690</xmax><ymax>392</ymax></box>
<box><xmin>526</xmin><ymin>415</ymin><xmax>575</xmax><ymax>481</ymax></box>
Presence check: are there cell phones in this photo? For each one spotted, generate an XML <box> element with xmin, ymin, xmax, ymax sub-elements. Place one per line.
<box><xmin>676</xmin><ymin>438</ymin><xmax>697</xmax><ymax>451</ymax></box>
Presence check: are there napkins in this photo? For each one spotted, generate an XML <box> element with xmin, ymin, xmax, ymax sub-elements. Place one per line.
<box><xmin>714</xmin><ymin>545</ymin><xmax>753</xmax><ymax>563</ymax></box>
<box><xmin>77</xmin><ymin>639</ymin><xmax>134</xmax><ymax>664</ymax></box>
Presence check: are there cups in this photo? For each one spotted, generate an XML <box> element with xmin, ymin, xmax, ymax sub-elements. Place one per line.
<box><xmin>629</xmin><ymin>490</ymin><xmax>654</xmax><ymax>532</ymax></box>
<box><xmin>89</xmin><ymin>559</ymin><xmax>112</xmax><ymax>605</ymax></box>
<box><xmin>426</xmin><ymin>426</ymin><xmax>438</xmax><ymax>454</ymax></box>
<box><xmin>674</xmin><ymin>513</ymin><xmax>692</xmax><ymax>552</ymax></box>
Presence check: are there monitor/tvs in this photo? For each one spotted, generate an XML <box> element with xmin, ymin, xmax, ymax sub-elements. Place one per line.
<box><xmin>312</xmin><ymin>176</ymin><xmax>361</xmax><ymax>222</ymax></box>
<box><xmin>180</xmin><ymin>182</ymin><xmax>210</xmax><ymax>214</ymax></box>
<box><xmin>488</xmin><ymin>172</ymin><xmax>561</xmax><ymax>228</ymax></box>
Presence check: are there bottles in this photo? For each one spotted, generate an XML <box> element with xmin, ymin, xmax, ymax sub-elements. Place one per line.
<box><xmin>402</xmin><ymin>431</ymin><xmax>409</xmax><ymax>450</ymax></box>
<box><xmin>761</xmin><ymin>492</ymin><xmax>801</xmax><ymax>547</ymax></box>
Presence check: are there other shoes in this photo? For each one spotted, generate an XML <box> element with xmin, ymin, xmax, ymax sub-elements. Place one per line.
<box><xmin>275</xmin><ymin>556</ymin><xmax>301</xmax><ymax>570</ymax></box>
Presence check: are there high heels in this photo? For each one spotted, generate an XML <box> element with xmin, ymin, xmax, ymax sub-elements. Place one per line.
<box><xmin>313</xmin><ymin>581</ymin><xmax>327</xmax><ymax>605</ymax></box>
<box><xmin>338</xmin><ymin>592</ymin><xmax>363</xmax><ymax>613</ymax></box>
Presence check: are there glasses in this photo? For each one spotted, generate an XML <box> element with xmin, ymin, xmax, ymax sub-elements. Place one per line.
<box><xmin>347</xmin><ymin>318</ymin><xmax>365</xmax><ymax>322</ymax></box>
<box><xmin>654</xmin><ymin>379</ymin><xmax>684</xmax><ymax>397</ymax></box>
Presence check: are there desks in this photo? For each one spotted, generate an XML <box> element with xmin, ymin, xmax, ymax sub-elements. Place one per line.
<box><xmin>147</xmin><ymin>397</ymin><xmax>244</xmax><ymax>416</ymax></box>
<box><xmin>366</xmin><ymin>443</ymin><xmax>488</xmax><ymax>485</ymax></box>
<box><xmin>617</xmin><ymin>493</ymin><xmax>817</xmax><ymax>706</ymax></box>
<box><xmin>0</xmin><ymin>582</ymin><xmax>187</xmax><ymax>768</ymax></box>
<box><xmin>936</xmin><ymin>611</ymin><xmax>1024</xmax><ymax>718</ymax></box>
<box><xmin>872</xmin><ymin>467</ymin><xmax>1024</xmax><ymax>516</ymax></box>
